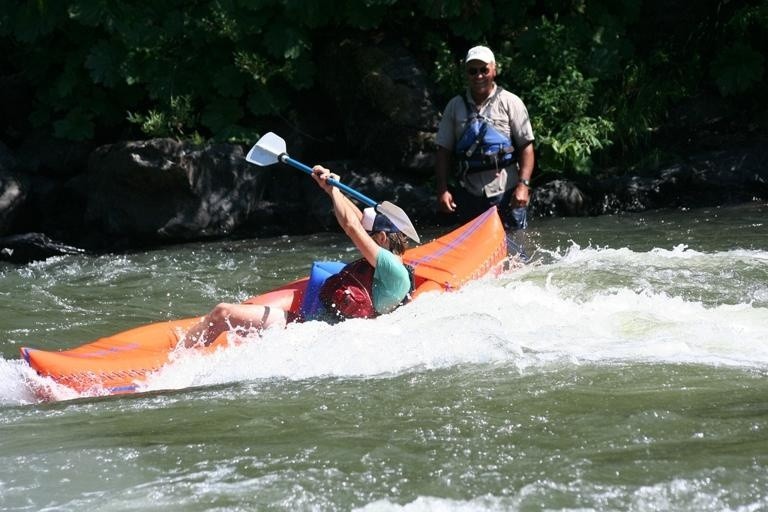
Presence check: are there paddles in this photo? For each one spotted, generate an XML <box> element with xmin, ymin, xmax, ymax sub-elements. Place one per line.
<box><xmin>245</xmin><ymin>132</ymin><xmax>421</xmax><ymax>244</ymax></box>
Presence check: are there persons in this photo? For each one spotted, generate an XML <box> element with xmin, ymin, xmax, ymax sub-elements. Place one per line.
<box><xmin>184</xmin><ymin>165</ymin><xmax>416</xmax><ymax>356</ymax></box>
<box><xmin>436</xmin><ymin>45</ymin><xmax>535</xmax><ymax>232</ymax></box>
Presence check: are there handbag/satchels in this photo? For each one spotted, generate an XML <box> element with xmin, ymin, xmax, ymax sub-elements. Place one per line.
<box><xmin>319</xmin><ymin>258</ymin><xmax>374</xmax><ymax>322</ymax></box>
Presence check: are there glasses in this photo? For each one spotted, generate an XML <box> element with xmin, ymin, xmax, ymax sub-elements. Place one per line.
<box><xmin>467</xmin><ymin>67</ymin><xmax>491</xmax><ymax>76</ymax></box>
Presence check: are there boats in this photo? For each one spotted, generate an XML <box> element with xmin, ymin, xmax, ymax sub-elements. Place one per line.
<box><xmin>19</xmin><ymin>205</ymin><xmax>510</xmax><ymax>400</ymax></box>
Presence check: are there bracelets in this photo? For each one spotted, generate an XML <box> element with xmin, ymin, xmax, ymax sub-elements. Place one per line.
<box><xmin>518</xmin><ymin>179</ymin><xmax>531</xmax><ymax>187</ymax></box>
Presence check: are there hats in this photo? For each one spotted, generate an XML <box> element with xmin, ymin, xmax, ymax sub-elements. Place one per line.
<box><xmin>360</xmin><ymin>201</ymin><xmax>420</xmax><ymax>244</ymax></box>
<box><xmin>465</xmin><ymin>45</ymin><xmax>495</xmax><ymax>64</ymax></box>
